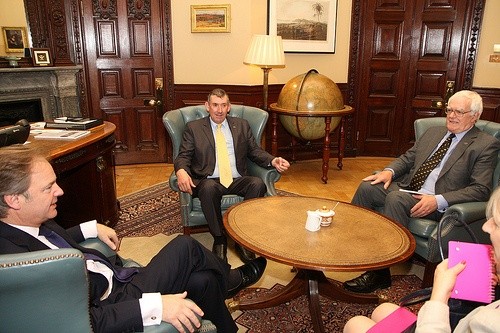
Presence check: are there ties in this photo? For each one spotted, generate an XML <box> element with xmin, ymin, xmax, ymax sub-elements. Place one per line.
<box><xmin>215</xmin><ymin>124</ymin><xmax>233</xmax><ymax>188</ymax></box>
<box><xmin>38</xmin><ymin>225</ymin><xmax>139</xmax><ymax>282</ymax></box>
<box><xmin>397</xmin><ymin>132</ymin><xmax>456</xmax><ymax>192</ymax></box>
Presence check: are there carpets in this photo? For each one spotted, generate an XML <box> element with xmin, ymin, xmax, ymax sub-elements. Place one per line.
<box><xmin>112</xmin><ymin>180</ymin><xmax>500</xmax><ymax>333</ymax></box>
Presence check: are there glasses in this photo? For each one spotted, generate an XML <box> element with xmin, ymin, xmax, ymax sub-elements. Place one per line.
<box><xmin>444</xmin><ymin>107</ymin><xmax>473</xmax><ymax>117</ymax></box>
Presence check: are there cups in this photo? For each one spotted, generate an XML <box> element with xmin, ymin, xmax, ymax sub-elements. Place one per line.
<box><xmin>316</xmin><ymin>206</ymin><xmax>335</xmax><ymax>226</ymax></box>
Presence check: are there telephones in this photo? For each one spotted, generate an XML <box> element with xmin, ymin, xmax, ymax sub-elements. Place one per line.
<box><xmin>0</xmin><ymin>119</ymin><xmax>31</xmax><ymax>148</ymax></box>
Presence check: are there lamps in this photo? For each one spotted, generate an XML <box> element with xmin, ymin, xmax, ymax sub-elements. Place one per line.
<box><xmin>242</xmin><ymin>34</ymin><xmax>286</xmax><ymax>110</ymax></box>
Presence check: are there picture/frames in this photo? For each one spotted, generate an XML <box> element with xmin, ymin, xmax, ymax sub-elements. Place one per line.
<box><xmin>267</xmin><ymin>0</ymin><xmax>339</xmax><ymax>53</ymax></box>
<box><xmin>32</xmin><ymin>48</ymin><xmax>53</xmax><ymax>66</ymax></box>
<box><xmin>2</xmin><ymin>26</ymin><xmax>28</xmax><ymax>53</ymax></box>
<box><xmin>190</xmin><ymin>4</ymin><xmax>231</xmax><ymax>33</ymax></box>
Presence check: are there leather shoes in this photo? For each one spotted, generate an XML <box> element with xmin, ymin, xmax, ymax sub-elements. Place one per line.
<box><xmin>225</xmin><ymin>256</ymin><xmax>268</xmax><ymax>298</ymax></box>
<box><xmin>344</xmin><ymin>268</ymin><xmax>392</xmax><ymax>293</ymax></box>
<box><xmin>212</xmin><ymin>235</ymin><xmax>228</xmax><ymax>260</ymax></box>
<box><xmin>235</xmin><ymin>242</ymin><xmax>256</xmax><ymax>263</ymax></box>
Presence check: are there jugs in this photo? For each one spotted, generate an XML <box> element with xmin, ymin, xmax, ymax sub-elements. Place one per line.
<box><xmin>305</xmin><ymin>210</ymin><xmax>322</xmax><ymax>231</ymax></box>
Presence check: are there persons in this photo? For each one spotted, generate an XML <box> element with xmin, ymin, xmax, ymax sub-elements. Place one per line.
<box><xmin>0</xmin><ymin>146</ymin><xmax>267</xmax><ymax>333</ymax></box>
<box><xmin>174</xmin><ymin>89</ymin><xmax>290</xmax><ymax>264</ymax></box>
<box><xmin>342</xmin><ymin>186</ymin><xmax>499</xmax><ymax>333</ymax></box>
<box><xmin>294</xmin><ymin>90</ymin><xmax>500</xmax><ymax>292</ymax></box>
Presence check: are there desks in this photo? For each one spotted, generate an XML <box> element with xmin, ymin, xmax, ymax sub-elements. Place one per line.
<box><xmin>0</xmin><ymin>120</ymin><xmax>120</xmax><ymax>228</ymax></box>
<box><xmin>227</xmin><ymin>195</ymin><xmax>416</xmax><ymax>333</ymax></box>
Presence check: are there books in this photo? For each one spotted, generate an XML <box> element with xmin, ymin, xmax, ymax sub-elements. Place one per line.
<box><xmin>34</xmin><ymin>129</ymin><xmax>90</xmax><ymax>138</ymax></box>
<box><xmin>447</xmin><ymin>240</ymin><xmax>494</xmax><ymax>304</ymax></box>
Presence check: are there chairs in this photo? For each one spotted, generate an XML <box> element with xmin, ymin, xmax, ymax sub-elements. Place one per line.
<box><xmin>405</xmin><ymin>117</ymin><xmax>500</xmax><ymax>289</ymax></box>
<box><xmin>0</xmin><ymin>238</ymin><xmax>216</xmax><ymax>333</ymax></box>
<box><xmin>164</xmin><ymin>104</ymin><xmax>280</xmax><ymax>239</ymax></box>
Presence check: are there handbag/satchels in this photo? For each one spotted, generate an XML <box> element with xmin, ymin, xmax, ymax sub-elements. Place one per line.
<box><xmin>399</xmin><ymin>214</ymin><xmax>500</xmax><ymax>333</ymax></box>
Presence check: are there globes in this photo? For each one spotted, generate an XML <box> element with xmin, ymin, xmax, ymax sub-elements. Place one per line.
<box><xmin>270</xmin><ymin>69</ymin><xmax>355</xmax><ymax>184</ymax></box>
